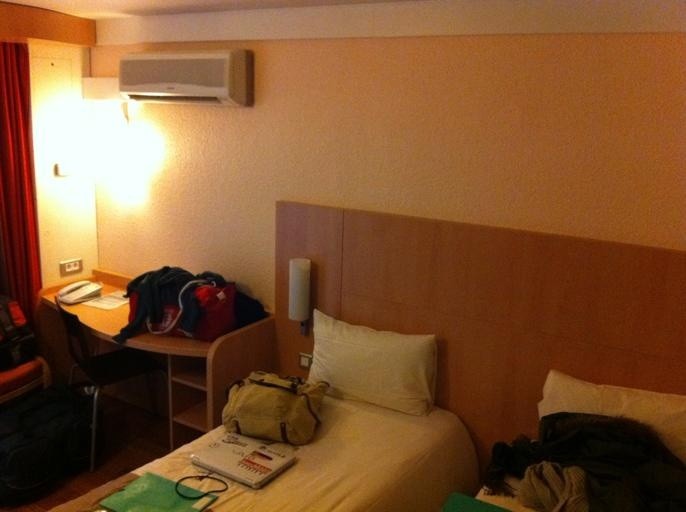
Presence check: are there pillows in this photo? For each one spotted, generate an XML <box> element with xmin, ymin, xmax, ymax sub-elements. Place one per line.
<box><xmin>539</xmin><ymin>368</ymin><xmax>685</xmax><ymax>464</ymax></box>
<box><xmin>305</xmin><ymin>308</ymin><xmax>437</xmax><ymax>418</ymax></box>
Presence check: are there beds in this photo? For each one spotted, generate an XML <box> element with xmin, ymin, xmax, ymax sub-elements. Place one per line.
<box><xmin>47</xmin><ymin>382</ymin><xmax>478</xmax><ymax>512</ymax></box>
<box><xmin>476</xmin><ymin>439</ymin><xmax>684</xmax><ymax>511</ymax></box>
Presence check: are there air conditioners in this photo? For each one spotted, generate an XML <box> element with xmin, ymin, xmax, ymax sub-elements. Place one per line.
<box><xmin>119</xmin><ymin>49</ymin><xmax>247</xmax><ymax>107</ymax></box>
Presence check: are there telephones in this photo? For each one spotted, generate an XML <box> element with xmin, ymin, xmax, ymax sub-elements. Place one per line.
<box><xmin>58</xmin><ymin>280</ymin><xmax>102</xmax><ymax>305</ymax></box>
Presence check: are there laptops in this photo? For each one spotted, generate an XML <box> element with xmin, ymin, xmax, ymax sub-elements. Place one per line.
<box><xmin>190</xmin><ymin>431</ymin><xmax>297</xmax><ymax>490</ymax></box>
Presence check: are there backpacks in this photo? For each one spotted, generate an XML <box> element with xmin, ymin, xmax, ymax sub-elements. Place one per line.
<box><xmin>0</xmin><ymin>288</ymin><xmax>38</xmax><ymax>371</ymax></box>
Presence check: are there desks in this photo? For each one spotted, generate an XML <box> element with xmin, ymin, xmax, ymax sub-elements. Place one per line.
<box><xmin>38</xmin><ymin>269</ymin><xmax>274</xmax><ymax>451</ymax></box>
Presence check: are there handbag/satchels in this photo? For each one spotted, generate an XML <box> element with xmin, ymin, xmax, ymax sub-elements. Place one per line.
<box><xmin>129</xmin><ymin>282</ymin><xmax>237</xmax><ymax>343</ymax></box>
<box><xmin>0</xmin><ymin>382</ymin><xmax>105</xmax><ymax>507</ymax></box>
<box><xmin>220</xmin><ymin>367</ymin><xmax>330</xmax><ymax>446</ymax></box>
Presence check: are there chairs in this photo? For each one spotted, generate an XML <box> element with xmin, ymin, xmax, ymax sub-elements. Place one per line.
<box><xmin>31</xmin><ymin>304</ymin><xmax>159</xmax><ymax>472</ymax></box>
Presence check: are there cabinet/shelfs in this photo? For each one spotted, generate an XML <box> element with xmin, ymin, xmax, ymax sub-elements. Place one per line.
<box><xmin>167</xmin><ymin>356</ymin><xmax>213</xmax><ymax>450</ymax></box>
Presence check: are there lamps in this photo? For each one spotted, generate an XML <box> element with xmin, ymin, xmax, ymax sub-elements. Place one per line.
<box><xmin>288</xmin><ymin>258</ymin><xmax>311</xmax><ymax>335</ymax></box>
